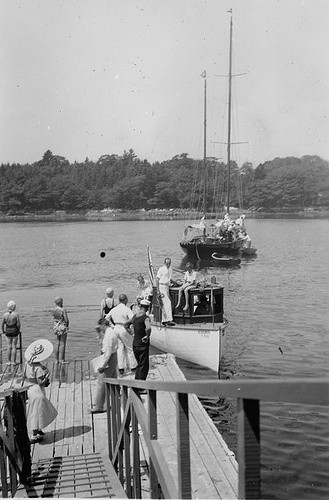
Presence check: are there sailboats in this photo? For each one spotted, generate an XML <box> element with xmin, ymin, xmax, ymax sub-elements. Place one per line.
<box><xmin>178</xmin><ymin>6</ymin><xmax>249</xmax><ymax>260</ymax></box>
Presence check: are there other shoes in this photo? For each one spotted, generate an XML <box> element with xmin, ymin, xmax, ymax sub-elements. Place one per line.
<box><xmin>31</xmin><ymin>437</ymin><xmax>43</xmax><ymax>444</ymax></box>
<box><xmin>33</xmin><ymin>430</ymin><xmax>45</xmax><ymax>436</ymax></box>
<box><xmin>90</xmin><ymin>406</ymin><xmax>104</xmax><ymax>413</ymax></box>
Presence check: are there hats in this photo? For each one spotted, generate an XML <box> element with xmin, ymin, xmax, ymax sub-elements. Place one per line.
<box><xmin>25</xmin><ymin>339</ymin><xmax>53</xmax><ymax>363</ymax></box>
<box><xmin>140</xmin><ymin>299</ymin><xmax>151</xmax><ymax>305</ymax></box>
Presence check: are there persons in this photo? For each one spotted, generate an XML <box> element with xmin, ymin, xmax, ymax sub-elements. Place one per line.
<box><xmin>51</xmin><ymin>297</ymin><xmax>71</xmax><ymax>364</ymax></box>
<box><xmin>175</xmin><ymin>262</ymin><xmax>199</xmax><ymax>311</ymax></box>
<box><xmin>155</xmin><ymin>258</ymin><xmax>180</xmax><ymax>328</ymax></box>
<box><xmin>131</xmin><ymin>300</ymin><xmax>156</xmax><ymax>395</ymax></box>
<box><xmin>101</xmin><ymin>289</ymin><xmax>118</xmax><ymax>318</ymax></box>
<box><xmin>215</xmin><ymin>213</ymin><xmax>252</xmax><ymax>250</ymax></box>
<box><xmin>22</xmin><ymin>340</ymin><xmax>57</xmax><ymax>443</ymax></box>
<box><xmin>88</xmin><ymin>318</ymin><xmax>119</xmax><ymax>413</ymax></box>
<box><xmin>133</xmin><ymin>277</ymin><xmax>153</xmax><ymax>313</ymax></box>
<box><xmin>105</xmin><ymin>294</ymin><xmax>139</xmax><ymax>376</ymax></box>
<box><xmin>1</xmin><ymin>301</ymin><xmax>19</xmax><ymax>366</ymax></box>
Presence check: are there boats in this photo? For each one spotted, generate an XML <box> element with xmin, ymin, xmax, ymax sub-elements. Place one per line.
<box><xmin>124</xmin><ymin>246</ymin><xmax>230</xmax><ymax>381</ymax></box>
<box><xmin>211</xmin><ymin>251</ymin><xmax>242</xmax><ymax>266</ymax></box>
<box><xmin>241</xmin><ymin>244</ymin><xmax>258</xmax><ymax>256</ymax></box>
